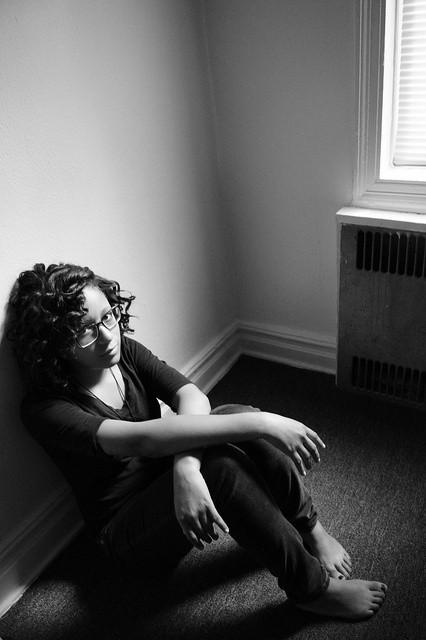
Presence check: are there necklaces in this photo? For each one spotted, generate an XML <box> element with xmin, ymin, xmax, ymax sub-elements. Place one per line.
<box><xmin>73</xmin><ymin>366</ymin><xmax>138</xmax><ymax>423</ymax></box>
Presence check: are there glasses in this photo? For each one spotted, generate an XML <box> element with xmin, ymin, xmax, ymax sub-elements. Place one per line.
<box><xmin>72</xmin><ymin>302</ymin><xmax>122</xmax><ymax>348</ymax></box>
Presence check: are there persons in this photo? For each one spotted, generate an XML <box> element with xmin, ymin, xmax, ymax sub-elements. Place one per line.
<box><xmin>6</xmin><ymin>262</ymin><xmax>388</xmax><ymax>619</ymax></box>
